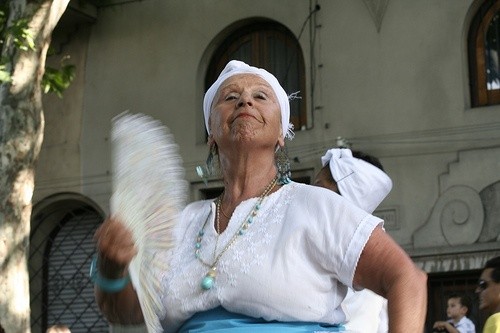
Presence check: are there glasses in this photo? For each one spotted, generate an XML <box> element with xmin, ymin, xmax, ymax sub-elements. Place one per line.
<box><xmin>479</xmin><ymin>278</ymin><xmax>500</xmax><ymax>290</ymax></box>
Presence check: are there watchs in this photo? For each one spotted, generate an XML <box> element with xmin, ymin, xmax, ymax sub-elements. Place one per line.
<box><xmin>90</xmin><ymin>254</ymin><xmax>130</xmax><ymax>290</ymax></box>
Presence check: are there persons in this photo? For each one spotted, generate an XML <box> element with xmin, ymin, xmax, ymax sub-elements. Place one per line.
<box><xmin>434</xmin><ymin>295</ymin><xmax>475</xmax><ymax>333</ymax></box>
<box><xmin>46</xmin><ymin>325</ymin><xmax>71</xmax><ymax>333</ymax></box>
<box><xmin>476</xmin><ymin>256</ymin><xmax>500</xmax><ymax>333</ymax></box>
<box><xmin>91</xmin><ymin>58</ymin><xmax>428</xmax><ymax>333</ymax></box>
<box><xmin>312</xmin><ymin>147</ymin><xmax>393</xmax><ymax>333</ymax></box>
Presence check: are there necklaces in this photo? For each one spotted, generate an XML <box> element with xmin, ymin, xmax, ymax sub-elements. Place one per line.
<box><xmin>194</xmin><ymin>172</ymin><xmax>282</xmax><ymax>290</ymax></box>
